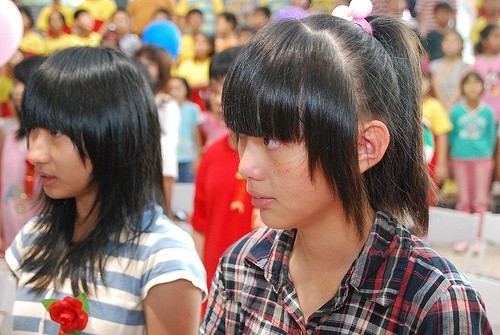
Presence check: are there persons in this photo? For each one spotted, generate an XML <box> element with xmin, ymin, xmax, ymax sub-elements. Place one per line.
<box><xmin>189</xmin><ymin>44</ymin><xmax>269</xmax><ymax>328</ymax></box>
<box><xmin>0</xmin><ymin>0</ymin><xmax>500</xmax><ymax>261</ymax></box>
<box><xmin>5</xmin><ymin>46</ymin><xmax>209</xmax><ymax>335</ymax></box>
<box><xmin>197</xmin><ymin>13</ymin><xmax>494</xmax><ymax>335</ymax></box>
